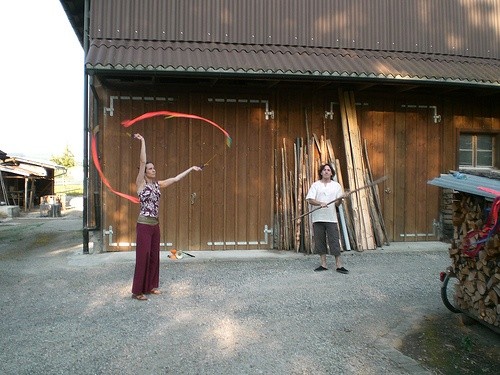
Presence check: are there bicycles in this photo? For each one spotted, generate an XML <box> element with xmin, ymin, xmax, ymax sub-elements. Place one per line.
<box><xmin>440</xmin><ymin>267</ymin><xmax>462</xmax><ymax>313</ymax></box>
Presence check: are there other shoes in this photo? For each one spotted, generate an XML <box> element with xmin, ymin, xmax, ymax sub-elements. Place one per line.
<box><xmin>314</xmin><ymin>264</ymin><xmax>328</xmax><ymax>272</ymax></box>
<box><xmin>336</xmin><ymin>267</ymin><xmax>349</xmax><ymax>273</ymax></box>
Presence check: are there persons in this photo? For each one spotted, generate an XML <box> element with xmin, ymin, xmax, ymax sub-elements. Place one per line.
<box><xmin>131</xmin><ymin>133</ymin><xmax>202</xmax><ymax>301</ymax></box>
<box><xmin>305</xmin><ymin>164</ymin><xmax>350</xmax><ymax>274</ymax></box>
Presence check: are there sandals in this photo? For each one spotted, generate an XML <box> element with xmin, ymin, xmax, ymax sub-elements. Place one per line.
<box><xmin>131</xmin><ymin>293</ymin><xmax>147</xmax><ymax>300</ymax></box>
<box><xmin>144</xmin><ymin>288</ymin><xmax>160</xmax><ymax>294</ymax></box>
